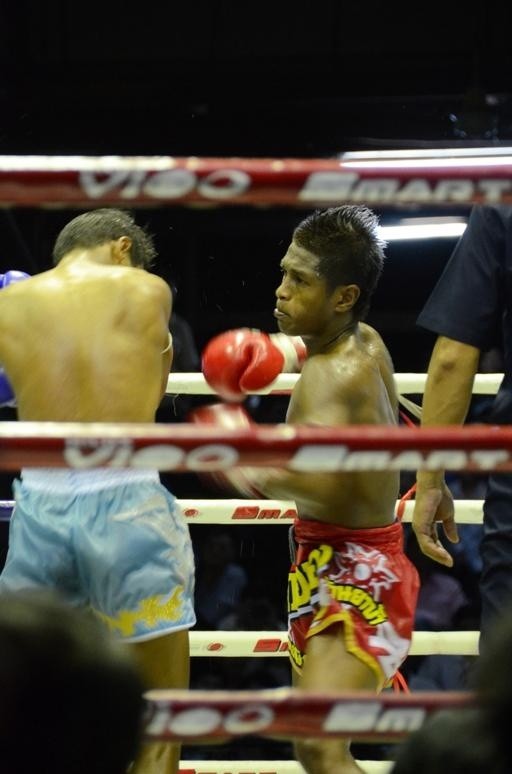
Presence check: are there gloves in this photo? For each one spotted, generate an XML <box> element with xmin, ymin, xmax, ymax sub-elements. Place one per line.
<box><xmin>200</xmin><ymin>326</ymin><xmax>305</xmax><ymax>401</ymax></box>
<box><xmin>182</xmin><ymin>401</ymin><xmax>279</xmax><ymax>497</ymax></box>
<box><xmin>0</xmin><ymin>369</ymin><xmax>16</xmax><ymax>409</ymax></box>
<box><xmin>0</xmin><ymin>268</ymin><xmax>31</xmax><ymax>288</ymax></box>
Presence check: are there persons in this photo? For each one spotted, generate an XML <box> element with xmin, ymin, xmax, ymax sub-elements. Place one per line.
<box><xmin>0</xmin><ymin>576</ymin><xmax>158</xmax><ymax>773</ymax></box>
<box><xmin>192</xmin><ymin>195</ymin><xmax>429</xmax><ymax>773</ymax></box>
<box><xmin>2</xmin><ymin>378</ymin><xmax>511</xmax><ymax>763</ymax></box>
<box><xmin>0</xmin><ymin>206</ymin><xmax>202</xmax><ymax>772</ymax></box>
<box><xmin>413</xmin><ymin>195</ymin><xmax>511</xmax><ymax>708</ymax></box>
<box><xmin>392</xmin><ymin>637</ymin><xmax>511</xmax><ymax>773</ymax></box>
